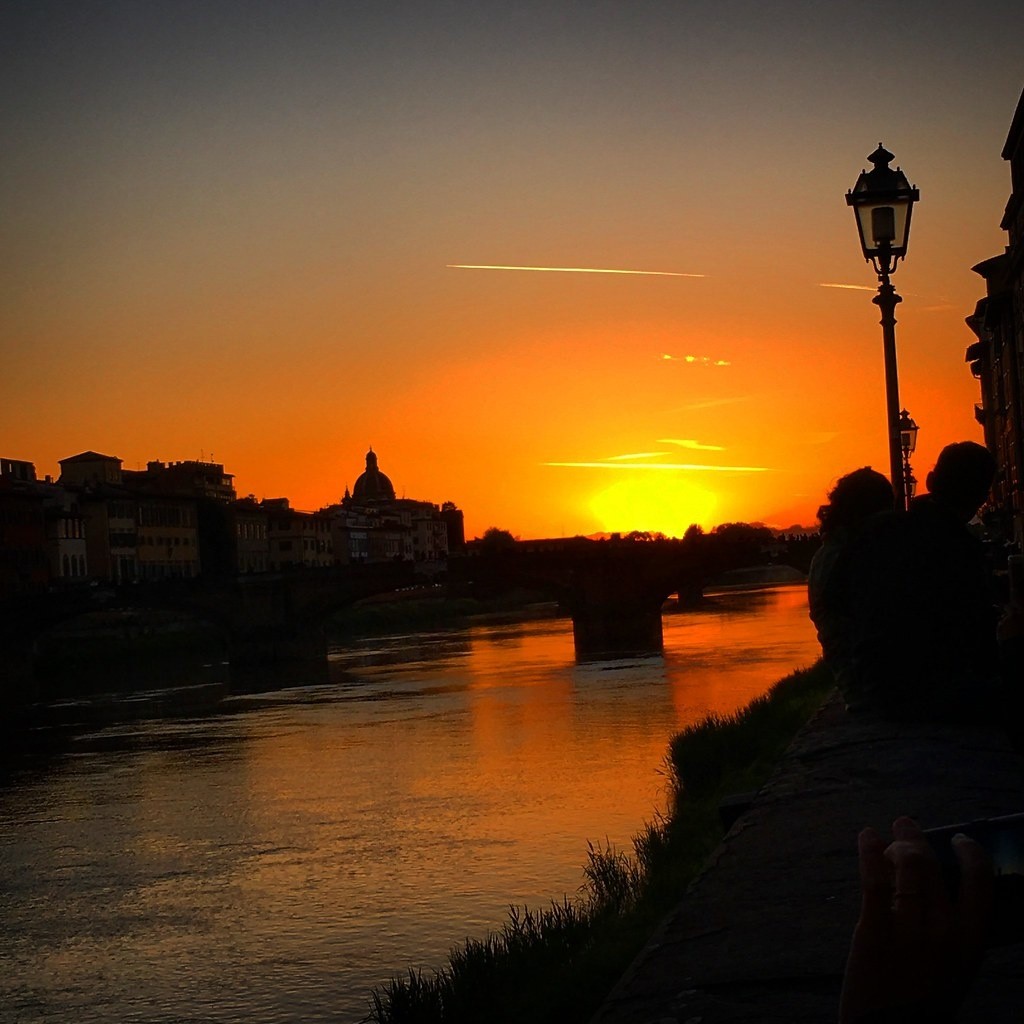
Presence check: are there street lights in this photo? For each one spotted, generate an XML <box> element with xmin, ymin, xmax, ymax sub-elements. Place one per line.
<box><xmin>899</xmin><ymin>408</ymin><xmax>917</xmax><ymax>509</ymax></box>
<box><xmin>846</xmin><ymin>142</ymin><xmax>920</xmax><ymax>508</ymax></box>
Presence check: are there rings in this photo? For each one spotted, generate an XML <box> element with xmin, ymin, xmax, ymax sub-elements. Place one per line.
<box><xmin>895</xmin><ymin>890</ymin><xmax>917</xmax><ymax>896</ymax></box>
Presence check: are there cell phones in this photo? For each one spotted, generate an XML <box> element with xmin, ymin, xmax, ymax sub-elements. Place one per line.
<box><xmin>1008</xmin><ymin>556</ymin><xmax>1024</xmax><ymax>602</ymax></box>
<box><xmin>917</xmin><ymin>813</ymin><xmax>1024</xmax><ymax>951</ymax></box>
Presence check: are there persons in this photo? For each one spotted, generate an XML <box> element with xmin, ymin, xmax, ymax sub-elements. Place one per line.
<box><xmin>812</xmin><ymin>442</ymin><xmax>1012</xmax><ymax>696</ymax></box>
<box><xmin>840</xmin><ymin>817</ymin><xmax>982</xmax><ymax>1024</ymax></box>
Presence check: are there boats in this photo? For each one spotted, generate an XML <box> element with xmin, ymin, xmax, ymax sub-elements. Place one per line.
<box><xmin>26</xmin><ymin>683</ymin><xmax>225</xmax><ymax>730</ymax></box>
